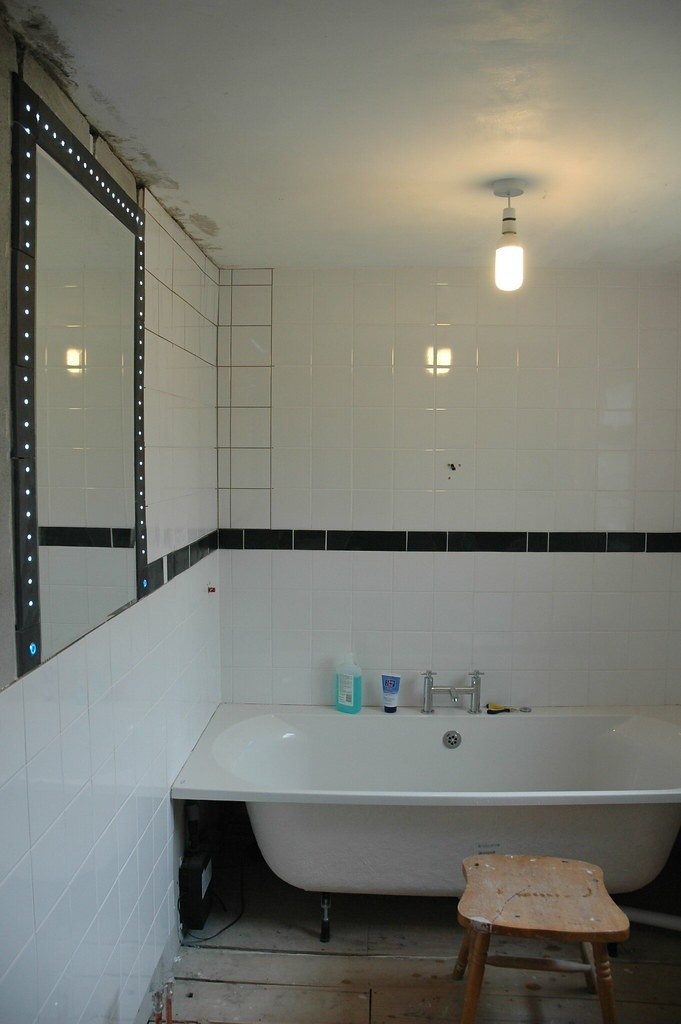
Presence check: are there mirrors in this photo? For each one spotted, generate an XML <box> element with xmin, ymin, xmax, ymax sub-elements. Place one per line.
<box><xmin>12</xmin><ymin>78</ymin><xmax>148</xmax><ymax>677</ymax></box>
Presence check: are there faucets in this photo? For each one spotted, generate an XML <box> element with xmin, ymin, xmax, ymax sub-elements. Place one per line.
<box><xmin>421</xmin><ymin>670</ymin><xmax>484</xmax><ymax>715</ymax></box>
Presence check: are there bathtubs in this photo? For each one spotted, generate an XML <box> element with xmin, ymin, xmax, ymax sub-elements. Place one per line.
<box><xmin>171</xmin><ymin>701</ymin><xmax>681</xmax><ymax>942</ymax></box>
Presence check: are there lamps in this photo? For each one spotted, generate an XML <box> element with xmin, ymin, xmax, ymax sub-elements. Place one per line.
<box><xmin>491</xmin><ymin>178</ymin><xmax>533</xmax><ymax>292</ymax></box>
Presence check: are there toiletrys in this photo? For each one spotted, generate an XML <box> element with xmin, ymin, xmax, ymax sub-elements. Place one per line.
<box><xmin>334</xmin><ymin>652</ymin><xmax>362</xmax><ymax>714</ymax></box>
<box><xmin>382</xmin><ymin>674</ymin><xmax>401</xmax><ymax>714</ymax></box>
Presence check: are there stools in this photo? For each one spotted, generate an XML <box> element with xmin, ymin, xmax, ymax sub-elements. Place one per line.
<box><xmin>450</xmin><ymin>853</ymin><xmax>631</xmax><ymax>1024</ymax></box>
